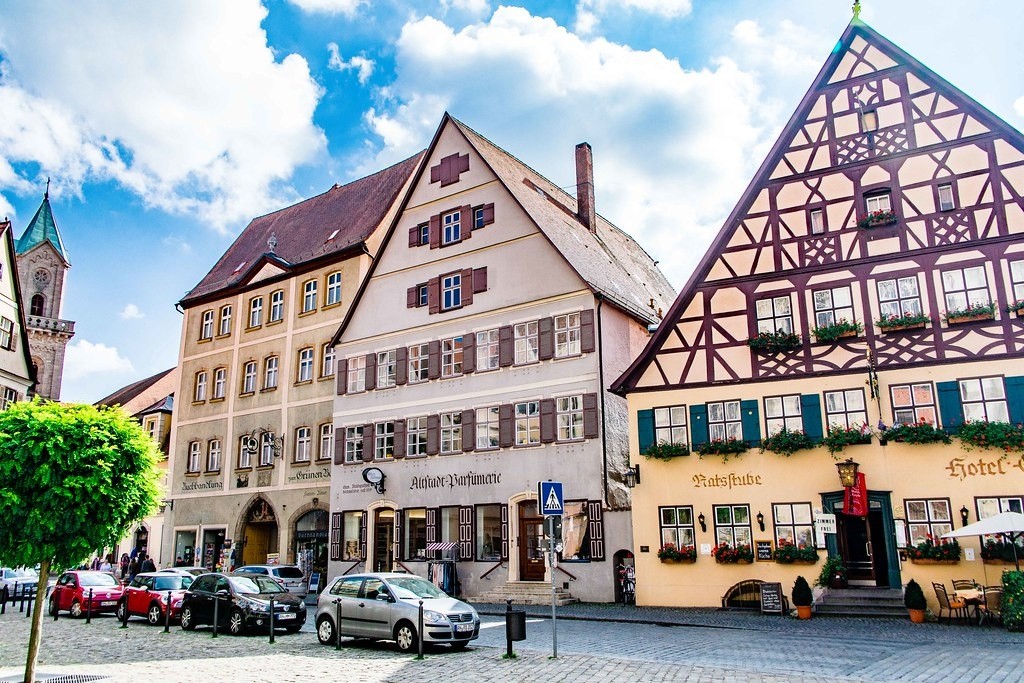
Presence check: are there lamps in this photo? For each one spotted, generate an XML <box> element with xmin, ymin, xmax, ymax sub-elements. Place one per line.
<box><xmin>757</xmin><ymin>511</ymin><xmax>765</xmax><ymax>531</ymax></box>
<box><xmin>698</xmin><ymin>512</ymin><xmax>706</xmax><ymax>532</ymax></box>
<box><xmin>835</xmin><ymin>459</ymin><xmax>860</xmax><ymax>487</ymax></box>
<box><xmin>960</xmin><ymin>505</ymin><xmax>969</xmax><ymax>527</ymax></box>
<box><xmin>623</xmin><ymin>469</ymin><xmax>636</xmax><ymax>488</ymax></box>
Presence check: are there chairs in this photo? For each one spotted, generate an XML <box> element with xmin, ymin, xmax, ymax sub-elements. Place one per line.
<box><xmin>932</xmin><ymin>582</ymin><xmax>966</xmax><ymax>626</ymax></box>
<box><xmin>983</xmin><ymin>585</ymin><xmax>1004</xmax><ymax>627</ymax></box>
<box><xmin>952</xmin><ymin>579</ymin><xmax>979</xmax><ymax>618</ymax></box>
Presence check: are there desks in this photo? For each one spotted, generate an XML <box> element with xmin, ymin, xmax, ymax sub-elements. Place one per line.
<box><xmin>954</xmin><ymin>589</ymin><xmax>984</xmax><ymax>627</ymax></box>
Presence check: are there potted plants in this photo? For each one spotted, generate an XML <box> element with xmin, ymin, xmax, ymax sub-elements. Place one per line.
<box><xmin>792</xmin><ymin>576</ymin><xmax>813</xmax><ymax>619</ymax></box>
<box><xmin>904</xmin><ymin>579</ymin><xmax>927</xmax><ymax>623</ymax></box>
<box><xmin>999</xmin><ymin>570</ymin><xmax>1024</xmax><ymax>632</ymax></box>
<box><xmin>819</xmin><ymin>554</ymin><xmax>848</xmax><ymax>589</ymax></box>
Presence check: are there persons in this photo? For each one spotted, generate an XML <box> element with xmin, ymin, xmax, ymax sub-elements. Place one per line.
<box><xmin>82</xmin><ymin>546</ymin><xmax>156</xmax><ymax>582</ymax></box>
<box><xmin>174</xmin><ymin>557</ymin><xmax>188</xmax><ymax>567</ymax></box>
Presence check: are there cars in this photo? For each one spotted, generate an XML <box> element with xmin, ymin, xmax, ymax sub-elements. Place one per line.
<box><xmin>179</xmin><ymin>572</ymin><xmax>307</xmax><ymax>636</ymax></box>
<box><xmin>314</xmin><ymin>573</ymin><xmax>480</xmax><ymax>654</ymax></box>
<box><xmin>148</xmin><ymin>566</ymin><xmax>211</xmax><ymax>589</ymax></box>
<box><xmin>49</xmin><ymin>571</ymin><xmax>125</xmax><ymax>618</ymax></box>
<box><xmin>230</xmin><ymin>564</ymin><xmax>308</xmax><ymax>600</ymax></box>
<box><xmin>115</xmin><ymin>572</ymin><xmax>195</xmax><ymax>626</ymax></box>
<box><xmin>0</xmin><ymin>567</ymin><xmax>52</xmax><ymax>597</ymax></box>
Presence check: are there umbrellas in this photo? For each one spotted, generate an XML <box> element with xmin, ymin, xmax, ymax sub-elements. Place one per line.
<box><xmin>940</xmin><ymin>509</ymin><xmax>1024</xmax><ymax>571</ymax></box>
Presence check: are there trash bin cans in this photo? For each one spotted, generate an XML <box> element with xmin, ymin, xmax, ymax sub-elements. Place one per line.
<box><xmin>506</xmin><ymin>610</ymin><xmax>526</xmax><ymax>642</ymax></box>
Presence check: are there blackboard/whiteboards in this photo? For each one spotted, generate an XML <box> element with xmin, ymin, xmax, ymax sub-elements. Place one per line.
<box><xmin>760</xmin><ymin>582</ymin><xmax>783</xmax><ymax>612</ymax></box>
<box><xmin>754</xmin><ymin>540</ymin><xmax>775</xmax><ymax>562</ymax></box>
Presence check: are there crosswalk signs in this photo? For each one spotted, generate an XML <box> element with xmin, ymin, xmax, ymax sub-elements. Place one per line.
<box><xmin>541</xmin><ymin>481</ymin><xmax>565</xmax><ymax>515</ymax></box>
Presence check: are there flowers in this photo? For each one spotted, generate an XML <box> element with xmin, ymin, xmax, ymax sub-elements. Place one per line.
<box><xmin>874</xmin><ymin>310</ymin><xmax>936</xmax><ymax>327</ymax></box>
<box><xmin>747</xmin><ymin>328</ymin><xmax>802</xmax><ymax>351</ymax></box>
<box><xmin>770</xmin><ymin>538</ymin><xmax>819</xmax><ymax>564</ymax></box>
<box><xmin>759</xmin><ymin>427</ymin><xmax>818</xmax><ymax>457</ymax></box>
<box><xmin>645</xmin><ymin>441</ymin><xmax>689</xmax><ymax>462</ymax></box>
<box><xmin>810</xmin><ymin>317</ymin><xmax>868</xmax><ymax>340</ymax></box>
<box><xmin>980</xmin><ymin>534</ymin><xmax>1024</xmax><ymax>562</ymax></box>
<box><xmin>853</xmin><ymin>209</ymin><xmax>898</xmax><ymax>229</ymax></box>
<box><xmin>940</xmin><ymin>302</ymin><xmax>995</xmax><ymax>318</ymax></box>
<box><xmin>818</xmin><ymin>422</ymin><xmax>873</xmax><ymax>451</ymax></box>
<box><xmin>875</xmin><ymin>417</ymin><xmax>953</xmax><ymax>445</ymax></box>
<box><xmin>906</xmin><ymin>533</ymin><xmax>962</xmax><ymax>561</ymax></box>
<box><xmin>711</xmin><ymin>542</ymin><xmax>754</xmax><ymax>563</ymax></box>
<box><xmin>1004</xmin><ymin>299</ymin><xmax>1024</xmax><ymax>313</ymax></box>
<box><xmin>657</xmin><ymin>543</ymin><xmax>697</xmax><ymax>562</ymax></box>
<box><xmin>698</xmin><ymin>436</ymin><xmax>751</xmax><ymax>463</ymax></box>
<box><xmin>955</xmin><ymin>420</ymin><xmax>1024</xmax><ymax>453</ymax></box>
